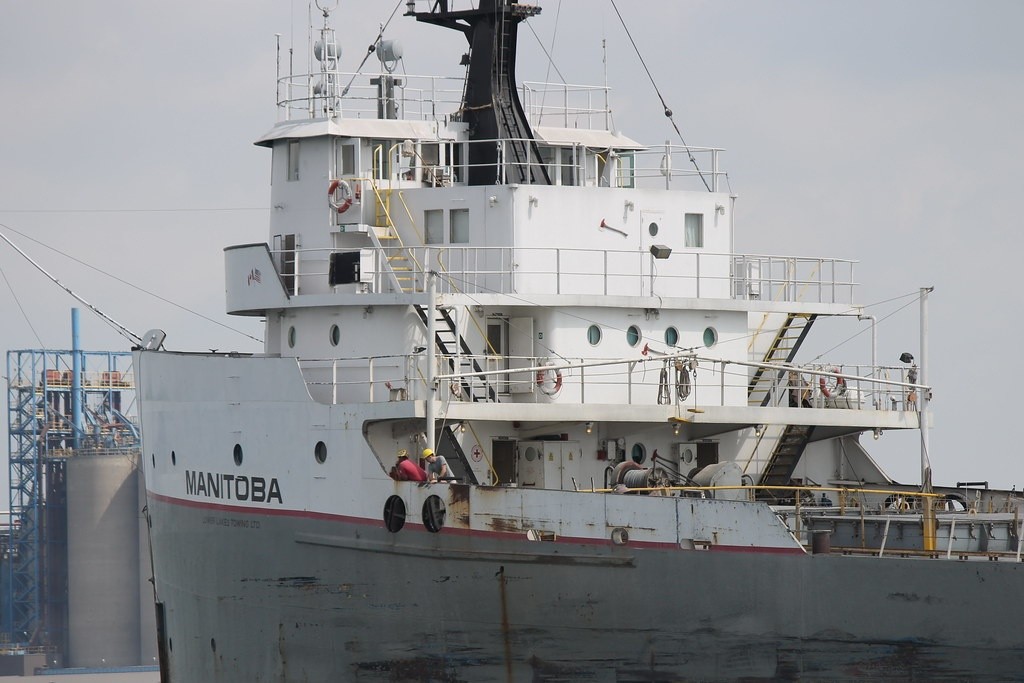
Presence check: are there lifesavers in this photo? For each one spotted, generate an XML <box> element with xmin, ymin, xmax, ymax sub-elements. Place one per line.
<box><xmin>536</xmin><ymin>362</ymin><xmax>563</xmax><ymax>395</ymax></box>
<box><xmin>819</xmin><ymin>367</ymin><xmax>844</xmax><ymax>397</ymax></box>
<box><xmin>327</xmin><ymin>181</ymin><xmax>352</xmax><ymax>212</ymax></box>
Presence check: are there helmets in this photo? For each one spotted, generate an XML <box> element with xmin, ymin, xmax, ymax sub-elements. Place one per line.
<box><xmin>397</xmin><ymin>448</ymin><xmax>409</xmax><ymax>459</ymax></box>
<box><xmin>423</xmin><ymin>449</ymin><xmax>433</xmax><ymax>458</ymax></box>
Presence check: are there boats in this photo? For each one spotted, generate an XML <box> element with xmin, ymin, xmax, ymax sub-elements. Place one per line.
<box><xmin>132</xmin><ymin>1</ymin><xmax>1024</xmax><ymax>683</ymax></box>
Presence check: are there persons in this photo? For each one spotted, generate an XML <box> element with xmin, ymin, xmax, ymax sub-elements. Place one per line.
<box><xmin>423</xmin><ymin>449</ymin><xmax>457</xmax><ymax>484</ymax></box>
<box><xmin>395</xmin><ymin>449</ymin><xmax>428</xmax><ymax>481</ymax></box>
